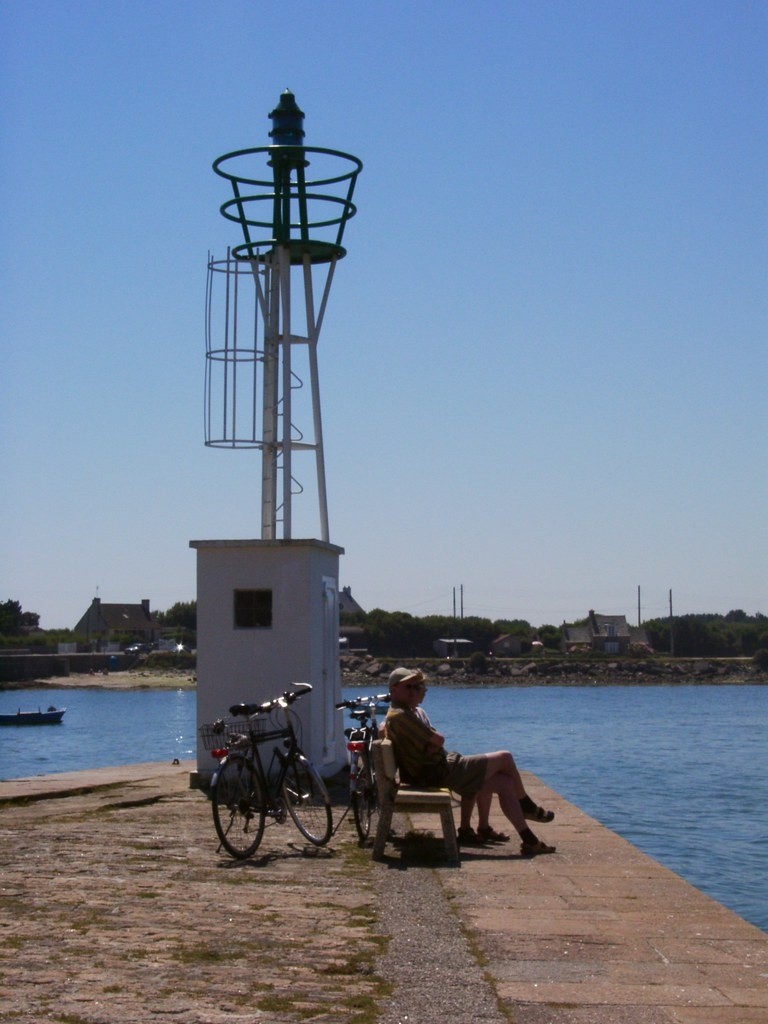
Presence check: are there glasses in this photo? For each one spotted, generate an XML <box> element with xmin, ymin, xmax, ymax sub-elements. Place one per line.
<box><xmin>404</xmin><ymin>684</ymin><xmax>416</xmax><ymax>689</ymax></box>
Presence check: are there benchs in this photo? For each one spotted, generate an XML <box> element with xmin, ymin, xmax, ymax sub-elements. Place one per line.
<box><xmin>371</xmin><ymin>738</ymin><xmax>461</xmax><ymax>861</ymax></box>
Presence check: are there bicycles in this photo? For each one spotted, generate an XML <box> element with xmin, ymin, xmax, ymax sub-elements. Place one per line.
<box><xmin>207</xmin><ymin>681</ymin><xmax>333</xmax><ymax>859</ymax></box>
<box><xmin>336</xmin><ymin>692</ymin><xmax>391</xmax><ymax>841</ymax></box>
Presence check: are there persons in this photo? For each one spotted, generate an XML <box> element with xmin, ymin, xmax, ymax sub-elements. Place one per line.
<box><xmin>387</xmin><ymin>668</ymin><xmax>556</xmax><ymax>854</ymax></box>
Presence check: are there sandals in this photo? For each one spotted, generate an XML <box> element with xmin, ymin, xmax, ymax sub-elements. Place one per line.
<box><xmin>524</xmin><ymin>807</ymin><xmax>554</xmax><ymax>822</ymax></box>
<box><xmin>521</xmin><ymin>841</ymin><xmax>556</xmax><ymax>853</ymax></box>
<box><xmin>458</xmin><ymin>828</ymin><xmax>493</xmax><ymax>844</ymax></box>
<box><xmin>477</xmin><ymin>826</ymin><xmax>510</xmax><ymax>841</ymax></box>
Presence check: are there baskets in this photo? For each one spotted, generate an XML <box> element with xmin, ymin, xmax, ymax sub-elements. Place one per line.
<box><xmin>198</xmin><ymin>719</ymin><xmax>267</xmax><ymax>750</ymax></box>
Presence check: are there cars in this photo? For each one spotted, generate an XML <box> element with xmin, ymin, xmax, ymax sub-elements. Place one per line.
<box><xmin>170</xmin><ymin>645</ymin><xmax>191</xmax><ymax>654</ymax></box>
<box><xmin>124</xmin><ymin>643</ymin><xmax>151</xmax><ymax>654</ymax></box>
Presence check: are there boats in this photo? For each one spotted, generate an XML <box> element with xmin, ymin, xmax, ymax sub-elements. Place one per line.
<box><xmin>0</xmin><ymin>707</ymin><xmax>66</xmax><ymax>724</ymax></box>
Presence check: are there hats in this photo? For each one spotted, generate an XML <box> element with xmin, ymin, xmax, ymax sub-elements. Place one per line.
<box><xmin>389</xmin><ymin>668</ymin><xmax>416</xmax><ymax>686</ymax></box>
